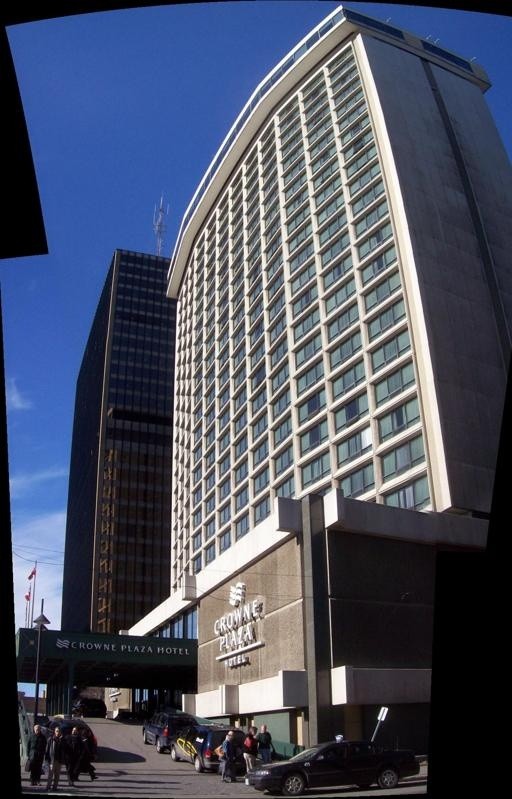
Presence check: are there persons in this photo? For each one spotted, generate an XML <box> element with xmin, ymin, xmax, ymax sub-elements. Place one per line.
<box><xmin>64</xmin><ymin>726</ymin><xmax>82</xmax><ymax>786</ymax></box>
<box><xmin>45</xmin><ymin>726</ymin><xmax>64</xmax><ymax>791</ymax></box>
<box><xmin>255</xmin><ymin>724</ymin><xmax>272</xmax><ymax>762</ymax></box>
<box><xmin>74</xmin><ymin>728</ymin><xmax>99</xmax><ymax>781</ymax></box>
<box><xmin>26</xmin><ymin>724</ymin><xmax>46</xmax><ymax>786</ymax></box>
<box><xmin>242</xmin><ymin>726</ymin><xmax>259</xmax><ymax>773</ymax></box>
<box><xmin>218</xmin><ymin>730</ymin><xmax>236</xmax><ymax>783</ymax></box>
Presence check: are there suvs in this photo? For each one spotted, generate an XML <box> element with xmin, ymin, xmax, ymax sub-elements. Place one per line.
<box><xmin>72</xmin><ymin>697</ymin><xmax>107</xmax><ymax>720</ymax></box>
<box><xmin>41</xmin><ymin>716</ymin><xmax>98</xmax><ymax>763</ymax></box>
<box><xmin>141</xmin><ymin>708</ymin><xmax>199</xmax><ymax>753</ymax></box>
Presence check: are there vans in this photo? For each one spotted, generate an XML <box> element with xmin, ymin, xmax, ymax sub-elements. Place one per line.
<box><xmin>121</xmin><ymin>711</ymin><xmax>140</xmax><ymax>725</ymax></box>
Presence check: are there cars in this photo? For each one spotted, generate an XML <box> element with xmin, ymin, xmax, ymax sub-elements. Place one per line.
<box><xmin>171</xmin><ymin>724</ymin><xmax>257</xmax><ymax>775</ymax></box>
<box><xmin>244</xmin><ymin>739</ymin><xmax>422</xmax><ymax>797</ymax></box>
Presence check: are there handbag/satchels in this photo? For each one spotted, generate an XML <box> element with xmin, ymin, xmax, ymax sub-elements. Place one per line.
<box><xmin>214</xmin><ymin>746</ymin><xmax>225</xmax><ymax>757</ymax></box>
<box><xmin>25</xmin><ymin>760</ymin><xmax>32</xmax><ymax>771</ymax></box>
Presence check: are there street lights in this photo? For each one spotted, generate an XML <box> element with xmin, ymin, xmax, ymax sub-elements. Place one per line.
<box><xmin>32</xmin><ymin>596</ymin><xmax>51</xmax><ymax>730</ymax></box>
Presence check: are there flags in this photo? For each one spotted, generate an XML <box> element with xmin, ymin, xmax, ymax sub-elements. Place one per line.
<box><xmin>28</xmin><ymin>566</ymin><xmax>37</xmax><ymax>580</ymax></box>
